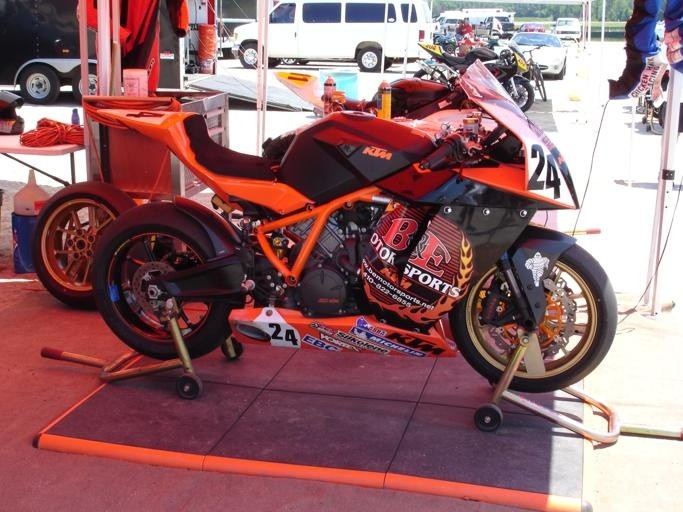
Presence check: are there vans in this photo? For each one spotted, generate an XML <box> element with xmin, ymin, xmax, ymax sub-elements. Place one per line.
<box><xmin>234</xmin><ymin>2</ymin><xmax>434</xmax><ymax>71</ymax></box>
<box><xmin>554</xmin><ymin>17</ymin><xmax>581</xmax><ymax>41</ymax></box>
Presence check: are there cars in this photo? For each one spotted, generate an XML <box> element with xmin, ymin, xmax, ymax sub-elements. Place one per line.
<box><xmin>437</xmin><ymin>9</ymin><xmax>516</xmax><ymax>37</ymax></box>
<box><xmin>511</xmin><ymin>27</ymin><xmax>567</xmax><ymax>79</ymax></box>
<box><xmin>521</xmin><ymin>23</ymin><xmax>545</xmax><ymax>32</ymax></box>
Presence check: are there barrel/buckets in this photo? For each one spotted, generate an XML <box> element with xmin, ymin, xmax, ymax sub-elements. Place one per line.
<box><xmin>199</xmin><ymin>24</ymin><xmax>215</xmax><ymax>73</ymax></box>
<box><xmin>12</xmin><ymin>215</ymin><xmax>38</xmax><ymax>275</ymax></box>
<box><xmin>14</xmin><ymin>169</ymin><xmax>48</xmax><ymax>215</ymax></box>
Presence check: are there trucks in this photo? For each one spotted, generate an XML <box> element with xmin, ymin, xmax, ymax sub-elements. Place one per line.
<box><xmin>1</xmin><ymin>0</ymin><xmax>219</xmax><ymax>106</ymax></box>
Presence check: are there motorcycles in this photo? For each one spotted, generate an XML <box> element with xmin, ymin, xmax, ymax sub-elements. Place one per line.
<box><xmin>92</xmin><ymin>57</ymin><xmax>619</xmax><ymax>392</ymax></box>
<box><xmin>274</xmin><ymin>28</ymin><xmax>534</xmax><ymax>120</ymax></box>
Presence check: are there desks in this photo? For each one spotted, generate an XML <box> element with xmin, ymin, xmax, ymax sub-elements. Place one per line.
<box><xmin>0</xmin><ymin>133</ymin><xmax>84</xmax><ymax>187</ymax></box>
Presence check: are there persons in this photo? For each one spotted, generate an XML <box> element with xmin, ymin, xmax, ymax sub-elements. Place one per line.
<box><xmin>455</xmin><ymin>19</ymin><xmax>463</xmax><ymax>36</ymax></box>
<box><xmin>459</xmin><ymin>16</ymin><xmax>472</xmax><ymax>34</ymax></box>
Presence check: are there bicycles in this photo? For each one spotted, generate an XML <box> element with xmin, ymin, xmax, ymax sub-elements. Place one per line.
<box><xmin>522</xmin><ymin>45</ymin><xmax>548</xmax><ymax>102</ymax></box>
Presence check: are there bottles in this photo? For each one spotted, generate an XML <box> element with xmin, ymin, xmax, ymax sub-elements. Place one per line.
<box><xmin>323</xmin><ymin>74</ymin><xmax>345</xmax><ymax>117</ymax></box>
<box><xmin>376</xmin><ymin>82</ymin><xmax>392</xmax><ymax>121</ymax></box>
